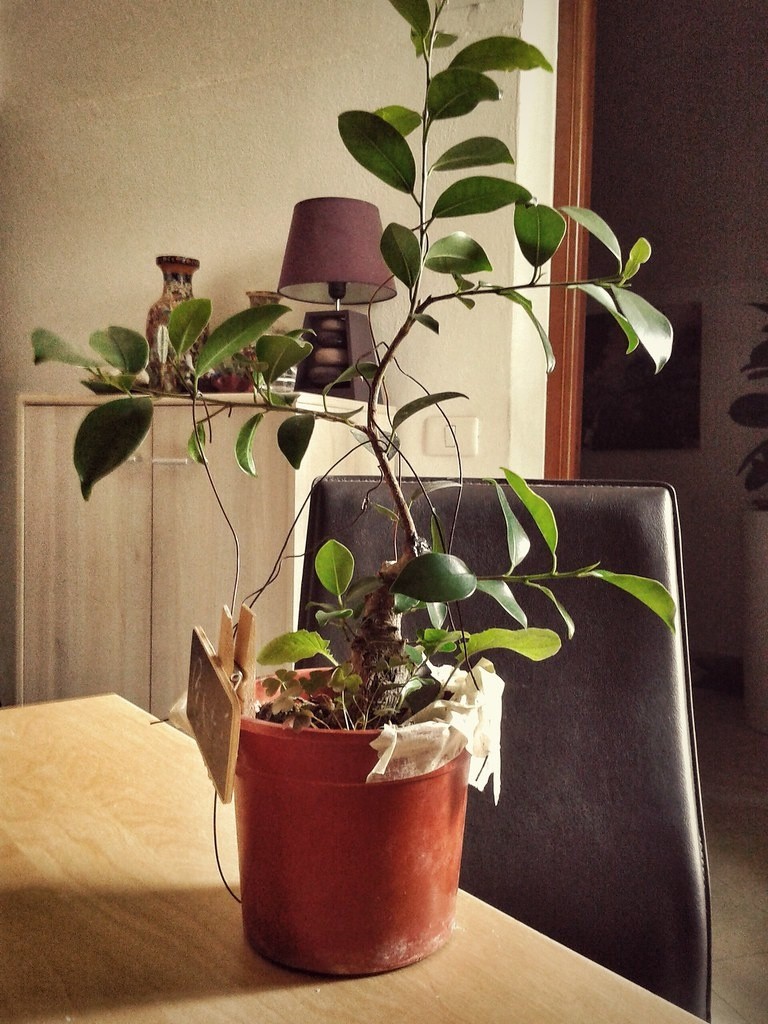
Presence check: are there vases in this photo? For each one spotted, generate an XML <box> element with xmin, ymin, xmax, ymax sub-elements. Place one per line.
<box><xmin>146</xmin><ymin>255</ymin><xmax>209</xmax><ymax>395</ymax></box>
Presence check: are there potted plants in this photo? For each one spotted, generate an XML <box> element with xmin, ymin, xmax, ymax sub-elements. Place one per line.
<box><xmin>727</xmin><ymin>301</ymin><xmax>768</xmax><ymax>737</ymax></box>
<box><xmin>29</xmin><ymin>0</ymin><xmax>674</xmax><ymax>976</ymax></box>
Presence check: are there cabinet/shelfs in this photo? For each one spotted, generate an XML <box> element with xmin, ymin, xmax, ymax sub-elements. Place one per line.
<box><xmin>14</xmin><ymin>390</ymin><xmax>397</xmax><ymax>738</ymax></box>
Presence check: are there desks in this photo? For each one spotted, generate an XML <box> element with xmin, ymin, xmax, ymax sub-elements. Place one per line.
<box><xmin>0</xmin><ymin>689</ymin><xmax>716</xmax><ymax>1023</ymax></box>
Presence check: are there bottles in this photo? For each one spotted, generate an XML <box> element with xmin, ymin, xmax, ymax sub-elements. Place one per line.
<box><xmin>145</xmin><ymin>255</ymin><xmax>208</xmax><ymax>393</ymax></box>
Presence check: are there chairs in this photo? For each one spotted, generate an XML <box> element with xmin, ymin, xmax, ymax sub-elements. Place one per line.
<box><xmin>291</xmin><ymin>474</ymin><xmax>713</xmax><ymax>1023</ymax></box>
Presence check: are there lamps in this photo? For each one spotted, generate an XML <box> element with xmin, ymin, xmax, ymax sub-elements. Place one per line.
<box><xmin>276</xmin><ymin>197</ymin><xmax>398</xmax><ymax>406</ymax></box>
